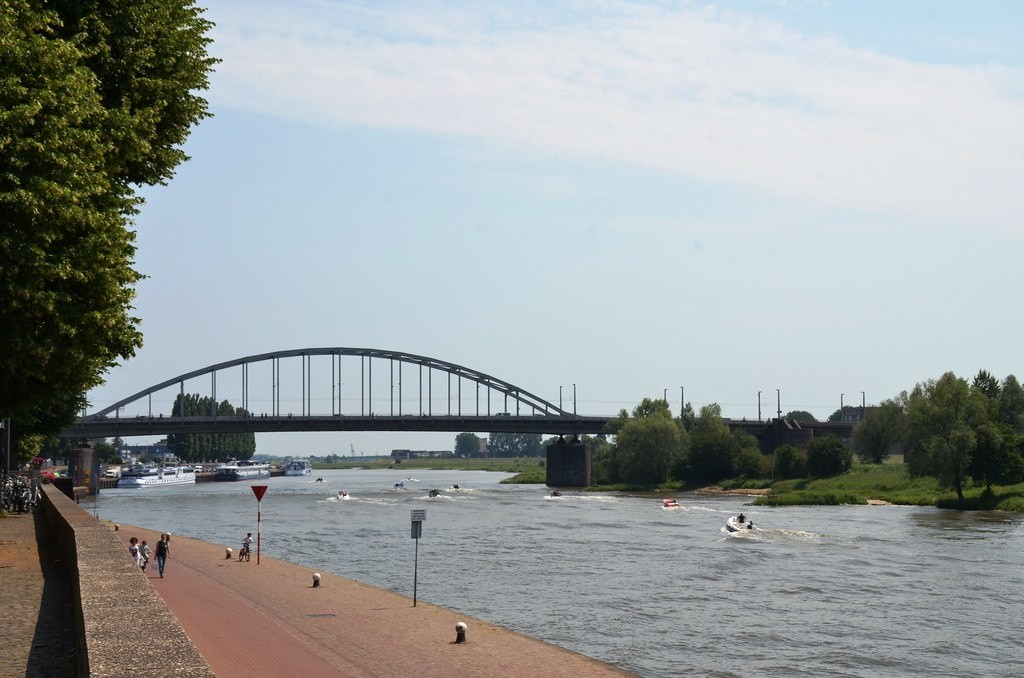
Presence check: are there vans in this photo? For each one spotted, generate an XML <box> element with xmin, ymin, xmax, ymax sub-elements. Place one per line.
<box><xmin>194</xmin><ymin>465</ymin><xmax>203</xmax><ymax>473</ymax></box>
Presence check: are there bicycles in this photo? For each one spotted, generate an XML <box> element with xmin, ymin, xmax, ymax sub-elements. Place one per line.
<box><xmin>0</xmin><ymin>470</ymin><xmax>44</xmax><ymax>513</ymax></box>
<box><xmin>239</xmin><ymin>541</ymin><xmax>253</xmax><ymax>562</ymax></box>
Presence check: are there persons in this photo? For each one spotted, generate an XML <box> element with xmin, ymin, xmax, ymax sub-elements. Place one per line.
<box><xmin>737</xmin><ymin>513</ymin><xmax>745</xmax><ymax>523</ymax></box>
<box><xmin>243</xmin><ymin>533</ymin><xmax>253</xmax><ymax>554</ymax></box>
<box><xmin>129</xmin><ymin>537</ymin><xmax>152</xmax><ymax>572</ymax></box>
<box><xmin>154</xmin><ymin>534</ymin><xmax>171</xmax><ymax>578</ymax></box>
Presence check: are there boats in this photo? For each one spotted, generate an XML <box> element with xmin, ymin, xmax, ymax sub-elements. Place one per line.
<box><xmin>662</xmin><ymin>499</ymin><xmax>680</xmax><ymax>508</ymax></box>
<box><xmin>283</xmin><ymin>459</ymin><xmax>312</xmax><ymax>477</ymax></box>
<box><xmin>117</xmin><ymin>467</ymin><xmax>195</xmax><ymax>488</ymax></box>
<box><xmin>338</xmin><ymin>490</ymin><xmax>350</xmax><ymax>501</ymax></box>
<box><xmin>216</xmin><ymin>459</ymin><xmax>271</xmax><ymax>482</ymax></box>
<box><xmin>725</xmin><ymin>515</ymin><xmax>761</xmax><ymax>534</ymax></box>
<box><xmin>428</xmin><ymin>489</ymin><xmax>446</xmax><ymax>498</ymax></box>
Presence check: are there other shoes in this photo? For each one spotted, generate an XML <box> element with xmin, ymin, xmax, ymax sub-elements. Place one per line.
<box><xmin>143</xmin><ymin>569</ymin><xmax>146</xmax><ymax>573</ymax></box>
<box><xmin>160</xmin><ymin>574</ymin><xmax>163</xmax><ymax>578</ymax></box>
